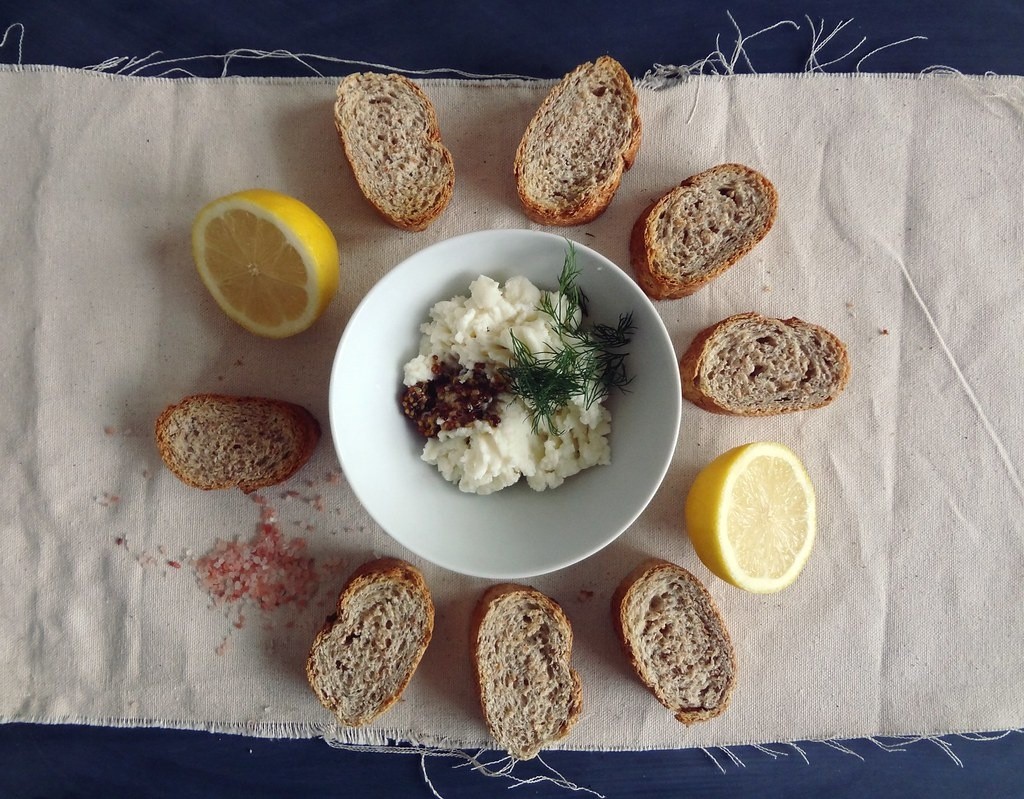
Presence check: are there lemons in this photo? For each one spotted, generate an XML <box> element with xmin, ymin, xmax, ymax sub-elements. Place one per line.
<box><xmin>191</xmin><ymin>188</ymin><xmax>340</xmax><ymax>339</ymax></box>
<box><xmin>685</xmin><ymin>439</ymin><xmax>815</xmax><ymax>596</ymax></box>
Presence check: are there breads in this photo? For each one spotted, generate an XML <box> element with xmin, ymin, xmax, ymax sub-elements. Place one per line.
<box><xmin>469</xmin><ymin>582</ymin><xmax>583</xmax><ymax>757</ymax></box>
<box><xmin>154</xmin><ymin>392</ymin><xmax>321</xmax><ymax>493</ymax></box>
<box><xmin>515</xmin><ymin>54</ymin><xmax>642</xmax><ymax>225</ymax></box>
<box><xmin>609</xmin><ymin>558</ymin><xmax>739</xmax><ymax>727</ymax></box>
<box><xmin>631</xmin><ymin>163</ymin><xmax>779</xmax><ymax>301</ymax></box>
<box><xmin>333</xmin><ymin>71</ymin><xmax>455</xmax><ymax>232</ymax></box>
<box><xmin>304</xmin><ymin>558</ymin><xmax>434</xmax><ymax>723</ymax></box>
<box><xmin>679</xmin><ymin>311</ymin><xmax>851</xmax><ymax>417</ymax></box>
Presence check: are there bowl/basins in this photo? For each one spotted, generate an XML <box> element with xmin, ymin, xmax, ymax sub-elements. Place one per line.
<box><xmin>327</xmin><ymin>227</ymin><xmax>681</xmax><ymax>581</ymax></box>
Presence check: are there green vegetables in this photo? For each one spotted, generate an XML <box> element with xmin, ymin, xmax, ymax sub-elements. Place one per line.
<box><xmin>500</xmin><ymin>233</ymin><xmax>642</xmax><ymax>435</ymax></box>
